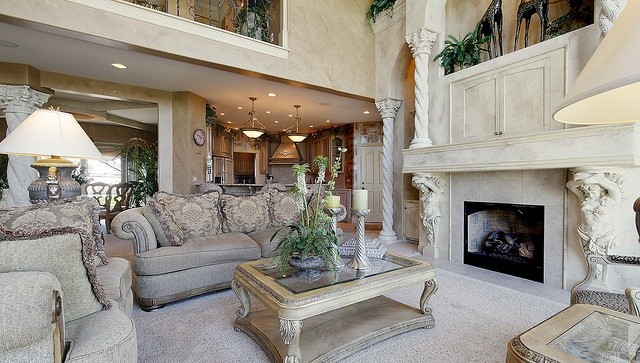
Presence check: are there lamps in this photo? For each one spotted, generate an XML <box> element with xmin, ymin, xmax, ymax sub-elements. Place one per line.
<box><xmin>0</xmin><ymin>103</ymin><xmax>104</xmax><ymax>204</ymax></box>
<box><xmin>552</xmin><ymin>2</ymin><xmax>639</xmax><ymax>245</ymax></box>
<box><xmin>238</xmin><ymin>93</ymin><xmax>266</xmax><ymax>142</ymax></box>
<box><xmin>284</xmin><ymin>103</ymin><xmax>310</xmax><ymax>145</ymax></box>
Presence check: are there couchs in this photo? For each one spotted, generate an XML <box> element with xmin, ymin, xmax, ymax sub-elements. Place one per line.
<box><xmin>0</xmin><ymin>251</ymin><xmax>140</xmax><ymax>363</ymax></box>
<box><xmin>108</xmin><ymin>190</ymin><xmax>306</xmax><ymax>313</ymax></box>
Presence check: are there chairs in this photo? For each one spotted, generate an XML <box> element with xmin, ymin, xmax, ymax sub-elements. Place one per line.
<box><xmin>98</xmin><ymin>181</ymin><xmax>134</xmax><ymax>237</ymax></box>
<box><xmin>84</xmin><ymin>182</ymin><xmax>111</xmax><ymax>230</ymax></box>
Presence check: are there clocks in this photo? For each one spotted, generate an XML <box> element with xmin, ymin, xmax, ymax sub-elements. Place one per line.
<box><xmin>192</xmin><ymin>127</ymin><xmax>208</xmax><ymax>147</ymax></box>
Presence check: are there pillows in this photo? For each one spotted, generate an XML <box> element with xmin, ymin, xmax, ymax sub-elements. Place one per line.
<box><xmin>0</xmin><ymin>230</ymin><xmax>112</xmax><ymax>325</ymax></box>
<box><xmin>152</xmin><ymin>189</ymin><xmax>222</xmax><ymax>238</ymax></box>
<box><xmin>0</xmin><ymin>195</ymin><xmax>112</xmax><ymax>269</ymax></box>
<box><xmin>267</xmin><ymin>189</ymin><xmax>301</xmax><ymax>230</ymax></box>
<box><xmin>143</xmin><ymin>199</ymin><xmax>184</xmax><ymax>247</ymax></box>
<box><xmin>218</xmin><ymin>192</ymin><xmax>272</xmax><ymax>234</ymax></box>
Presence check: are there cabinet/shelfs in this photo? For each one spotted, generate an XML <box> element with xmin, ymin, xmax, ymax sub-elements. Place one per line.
<box><xmin>446</xmin><ymin>48</ymin><xmax>567</xmax><ymax>142</ymax></box>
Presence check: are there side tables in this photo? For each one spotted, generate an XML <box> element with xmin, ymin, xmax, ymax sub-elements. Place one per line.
<box><xmin>506</xmin><ymin>300</ymin><xmax>638</xmax><ymax>362</ymax></box>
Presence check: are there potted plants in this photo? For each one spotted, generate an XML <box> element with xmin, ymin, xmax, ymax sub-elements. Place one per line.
<box><xmin>437</xmin><ymin>34</ymin><xmax>481</xmax><ymax>75</ymax></box>
<box><xmin>547</xmin><ymin>0</ymin><xmax>593</xmax><ymax>37</ymax></box>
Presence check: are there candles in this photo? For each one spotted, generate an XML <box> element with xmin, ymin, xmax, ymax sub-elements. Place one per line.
<box><xmin>350</xmin><ymin>187</ymin><xmax>370</xmax><ymax>211</ymax></box>
<box><xmin>323</xmin><ymin>193</ymin><xmax>342</xmax><ymax>209</ymax></box>
